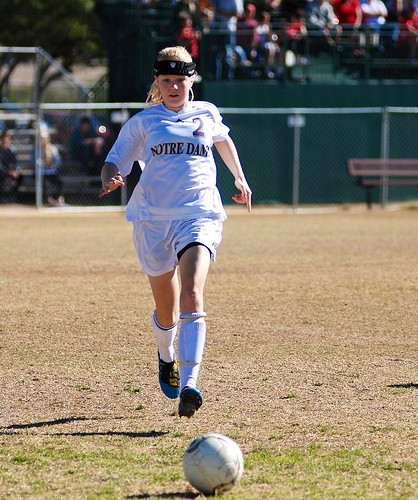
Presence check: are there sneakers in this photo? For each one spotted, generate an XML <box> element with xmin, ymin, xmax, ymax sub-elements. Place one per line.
<box><xmin>158</xmin><ymin>350</ymin><xmax>181</xmax><ymax>399</ymax></box>
<box><xmin>178</xmin><ymin>386</ymin><xmax>202</xmax><ymax>418</ymax></box>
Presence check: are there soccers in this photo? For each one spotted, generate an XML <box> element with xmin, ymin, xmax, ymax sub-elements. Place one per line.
<box><xmin>182</xmin><ymin>432</ymin><xmax>246</xmax><ymax>496</ymax></box>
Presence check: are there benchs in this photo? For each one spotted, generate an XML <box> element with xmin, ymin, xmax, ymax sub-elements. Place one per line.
<box><xmin>346</xmin><ymin>157</ymin><xmax>418</xmax><ymax>210</ymax></box>
<box><xmin>0</xmin><ymin>109</ymin><xmax>102</xmax><ymax>201</ymax></box>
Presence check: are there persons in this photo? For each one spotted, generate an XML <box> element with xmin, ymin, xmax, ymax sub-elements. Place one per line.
<box><xmin>100</xmin><ymin>46</ymin><xmax>252</xmax><ymax>417</ymax></box>
<box><xmin>29</xmin><ymin>132</ymin><xmax>67</xmax><ymax>207</ymax></box>
<box><xmin>71</xmin><ymin>116</ymin><xmax>104</xmax><ymax>167</ymax></box>
<box><xmin>166</xmin><ymin>0</ymin><xmax>417</xmax><ymax>84</ymax></box>
<box><xmin>0</xmin><ymin>133</ymin><xmax>20</xmax><ymax>204</ymax></box>
